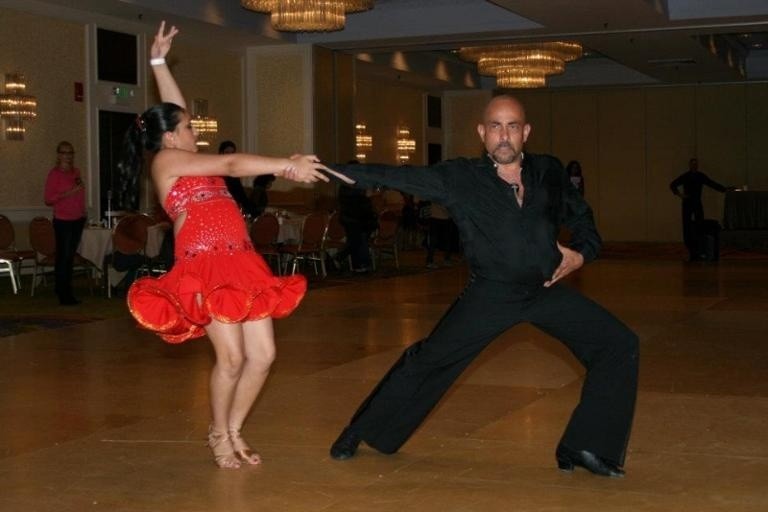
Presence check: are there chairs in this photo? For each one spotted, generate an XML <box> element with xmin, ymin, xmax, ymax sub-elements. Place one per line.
<box><xmin>0</xmin><ymin>208</ymin><xmax>173</xmax><ymax>298</ymax></box>
<box><xmin>250</xmin><ymin>190</ymin><xmax>437</xmax><ymax>279</ymax></box>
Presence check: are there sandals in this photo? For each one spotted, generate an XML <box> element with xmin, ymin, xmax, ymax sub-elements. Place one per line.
<box><xmin>229</xmin><ymin>424</ymin><xmax>261</xmax><ymax>466</ymax></box>
<box><xmin>205</xmin><ymin>423</ymin><xmax>242</xmax><ymax>470</ymax></box>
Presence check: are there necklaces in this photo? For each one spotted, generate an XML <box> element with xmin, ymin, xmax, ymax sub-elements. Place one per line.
<box><xmin>496</xmin><ymin>163</ymin><xmax>521</xmax><ymax>199</ymax></box>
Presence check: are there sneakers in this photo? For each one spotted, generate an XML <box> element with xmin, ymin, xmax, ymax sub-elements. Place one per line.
<box><xmin>60</xmin><ymin>299</ymin><xmax>82</xmax><ymax>305</ymax></box>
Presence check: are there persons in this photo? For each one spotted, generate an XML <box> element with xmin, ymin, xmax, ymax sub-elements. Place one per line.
<box><xmin>670</xmin><ymin>156</ymin><xmax>736</xmax><ymax>263</ymax></box>
<box><xmin>567</xmin><ymin>160</ymin><xmax>585</xmax><ymax>199</ymax></box>
<box><xmin>333</xmin><ymin>159</ymin><xmax>458</xmax><ymax>278</ymax></box>
<box><xmin>41</xmin><ymin>140</ymin><xmax>88</xmax><ymax>305</ymax></box>
<box><xmin>125</xmin><ymin>17</ymin><xmax>332</xmax><ymax>471</ymax></box>
<box><xmin>218</xmin><ymin>141</ymin><xmax>251</xmax><ymax>212</ymax></box>
<box><xmin>273</xmin><ymin>93</ymin><xmax>640</xmax><ymax>477</ymax></box>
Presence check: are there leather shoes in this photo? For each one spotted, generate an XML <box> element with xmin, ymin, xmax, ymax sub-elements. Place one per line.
<box><xmin>329</xmin><ymin>423</ymin><xmax>362</xmax><ymax>460</ymax></box>
<box><xmin>555</xmin><ymin>444</ymin><xmax>628</xmax><ymax>479</ymax></box>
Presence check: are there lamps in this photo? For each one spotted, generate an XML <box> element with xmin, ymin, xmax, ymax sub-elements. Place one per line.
<box><xmin>349</xmin><ymin>120</ymin><xmax>373</xmax><ymax>163</ymax></box>
<box><xmin>2</xmin><ymin>71</ymin><xmax>36</xmax><ymax>142</ymax></box>
<box><xmin>395</xmin><ymin>123</ymin><xmax>419</xmax><ymax>166</ymax></box>
<box><xmin>454</xmin><ymin>38</ymin><xmax>587</xmax><ymax>92</ymax></box>
<box><xmin>190</xmin><ymin>112</ymin><xmax>219</xmax><ymax>150</ymax></box>
<box><xmin>238</xmin><ymin>0</ymin><xmax>375</xmax><ymax>35</ymax></box>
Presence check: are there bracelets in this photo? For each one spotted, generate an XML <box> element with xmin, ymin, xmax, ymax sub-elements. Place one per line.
<box><xmin>149</xmin><ymin>57</ymin><xmax>166</xmax><ymax>66</ymax></box>
